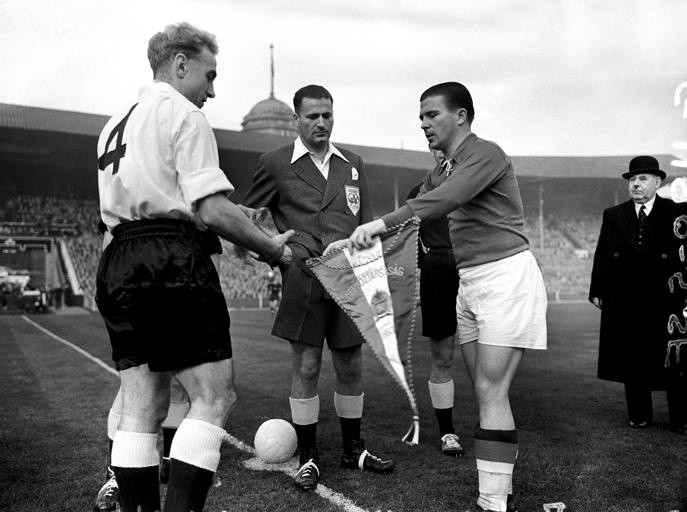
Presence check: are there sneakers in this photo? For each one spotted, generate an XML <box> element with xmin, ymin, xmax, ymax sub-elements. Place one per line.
<box><xmin>341</xmin><ymin>438</ymin><xmax>395</xmax><ymax>475</ymax></box>
<box><xmin>294</xmin><ymin>448</ymin><xmax>320</xmax><ymax>490</ymax></box>
<box><xmin>439</xmin><ymin>433</ymin><xmax>465</xmax><ymax>459</ymax></box>
<box><xmin>94</xmin><ymin>472</ymin><xmax>119</xmax><ymax>510</ymax></box>
<box><xmin>465</xmin><ymin>503</ymin><xmax>520</xmax><ymax>512</ymax></box>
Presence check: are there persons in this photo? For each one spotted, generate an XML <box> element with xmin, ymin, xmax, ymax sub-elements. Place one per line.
<box><xmin>0</xmin><ymin>22</ymin><xmax>687</xmax><ymax>512</ymax></box>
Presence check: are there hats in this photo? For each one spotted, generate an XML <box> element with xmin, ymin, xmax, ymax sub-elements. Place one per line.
<box><xmin>622</xmin><ymin>156</ymin><xmax>666</xmax><ymax>181</ymax></box>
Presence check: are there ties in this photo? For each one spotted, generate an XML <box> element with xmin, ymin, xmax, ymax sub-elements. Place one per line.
<box><xmin>639</xmin><ymin>205</ymin><xmax>647</xmax><ymax>221</ymax></box>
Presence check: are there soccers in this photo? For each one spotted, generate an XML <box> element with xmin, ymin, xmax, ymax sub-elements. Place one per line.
<box><xmin>253</xmin><ymin>419</ymin><xmax>297</xmax><ymax>463</ymax></box>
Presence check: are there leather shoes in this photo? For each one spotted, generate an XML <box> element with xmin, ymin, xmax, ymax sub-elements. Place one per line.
<box><xmin>629</xmin><ymin>418</ymin><xmax>687</xmax><ymax>436</ymax></box>
